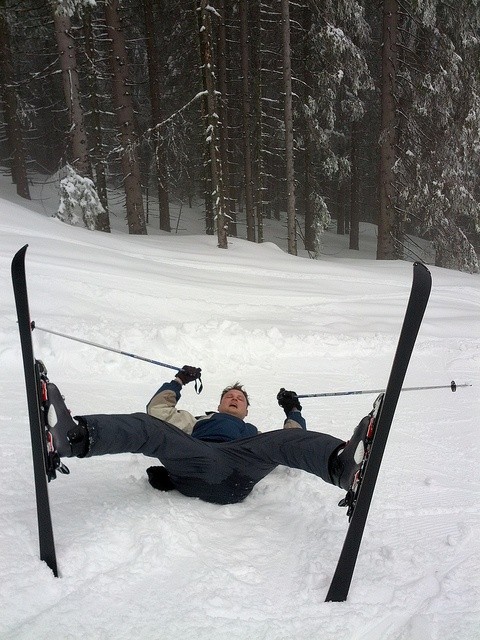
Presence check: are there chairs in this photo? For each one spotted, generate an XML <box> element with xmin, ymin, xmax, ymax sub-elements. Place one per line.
<box><xmin>45</xmin><ymin>383</ymin><xmax>75</xmax><ymax>459</ymax></box>
<box><xmin>341</xmin><ymin>418</ymin><xmax>370</xmax><ymax>489</ymax></box>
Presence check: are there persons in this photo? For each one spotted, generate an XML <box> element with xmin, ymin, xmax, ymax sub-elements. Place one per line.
<box><xmin>44</xmin><ymin>365</ymin><xmax>368</xmax><ymax>504</ymax></box>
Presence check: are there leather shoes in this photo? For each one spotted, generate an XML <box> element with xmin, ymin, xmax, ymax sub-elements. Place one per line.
<box><xmin>279</xmin><ymin>386</ymin><xmax>300</xmax><ymax>409</ymax></box>
<box><xmin>176</xmin><ymin>366</ymin><xmax>203</xmax><ymax>384</ymax></box>
